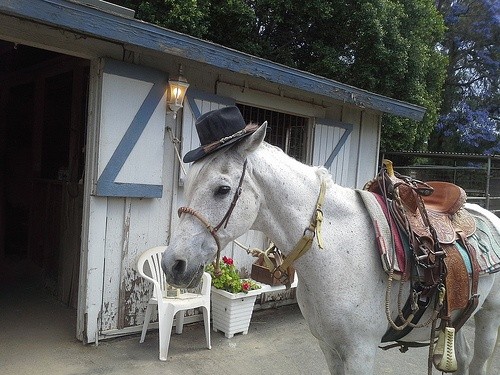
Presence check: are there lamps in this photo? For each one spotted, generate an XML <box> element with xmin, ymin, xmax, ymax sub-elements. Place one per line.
<box><xmin>167</xmin><ymin>64</ymin><xmax>191</xmax><ymax>120</ymax></box>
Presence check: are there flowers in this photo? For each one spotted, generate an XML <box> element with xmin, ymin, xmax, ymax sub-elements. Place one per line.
<box><xmin>204</xmin><ymin>255</ymin><xmax>262</xmax><ymax>295</ymax></box>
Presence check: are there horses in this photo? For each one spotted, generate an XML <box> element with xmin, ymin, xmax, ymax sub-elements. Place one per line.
<box><xmin>160</xmin><ymin>119</ymin><xmax>500</xmax><ymax>375</ymax></box>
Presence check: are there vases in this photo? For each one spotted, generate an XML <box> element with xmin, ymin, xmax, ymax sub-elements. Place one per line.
<box><xmin>211</xmin><ymin>279</ymin><xmax>264</xmax><ymax>339</ymax></box>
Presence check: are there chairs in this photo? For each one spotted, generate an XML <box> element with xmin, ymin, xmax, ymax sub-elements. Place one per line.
<box><xmin>137</xmin><ymin>246</ymin><xmax>212</xmax><ymax>361</ymax></box>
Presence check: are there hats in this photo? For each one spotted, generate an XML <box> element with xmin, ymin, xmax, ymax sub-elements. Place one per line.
<box><xmin>183</xmin><ymin>106</ymin><xmax>258</xmax><ymax>163</ymax></box>
